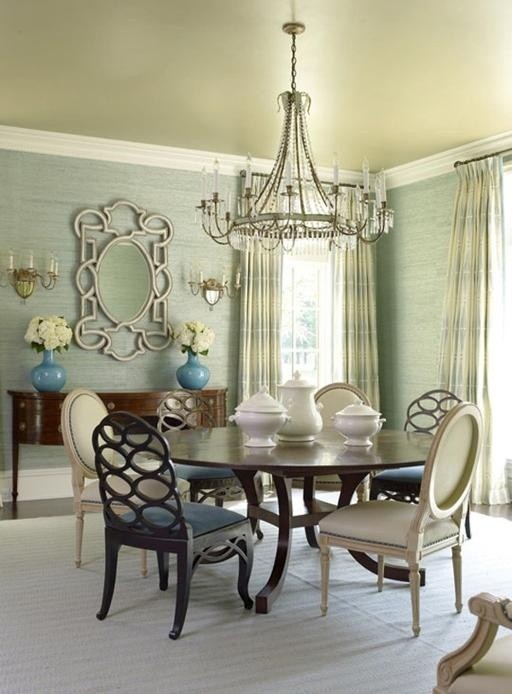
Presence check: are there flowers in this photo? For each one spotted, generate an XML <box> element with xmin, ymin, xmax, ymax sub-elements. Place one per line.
<box><xmin>175</xmin><ymin>319</ymin><xmax>215</xmax><ymax>356</ymax></box>
<box><xmin>24</xmin><ymin>315</ymin><xmax>72</xmax><ymax>353</ymax></box>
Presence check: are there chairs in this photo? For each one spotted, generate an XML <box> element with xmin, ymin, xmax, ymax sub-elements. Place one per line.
<box><xmin>368</xmin><ymin>390</ymin><xmax>474</xmax><ymax>540</ymax></box>
<box><xmin>314</xmin><ymin>381</ymin><xmax>372</xmax><ymax>502</ymax></box>
<box><xmin>319</xmin><ymin>403</ymin><xmax>483</xmax><ymax>636</ymax></box>
<box><xmin>61</xmin><ymin>389</ymin><xmax>192</xmax><ymax>578</ymax></box>
<box><xmin>156</xmin><ymin>388</ymin><xmax>247</xmax><ymax>506</ymax></box>
<box><xmin>91</xmin><ymin>411</ymin><xmax>255</xmax><ymax>639</ymax></box>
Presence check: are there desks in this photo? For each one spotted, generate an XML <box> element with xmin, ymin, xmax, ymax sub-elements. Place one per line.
<box><xmin>8</xmin><ymin>389</ymin><xmax>230</xmax><ymax>517</ymax></box>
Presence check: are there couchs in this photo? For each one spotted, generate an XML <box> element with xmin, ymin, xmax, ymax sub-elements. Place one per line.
<box><xmin>432</xmin><ymin>592</ymin><xmax>512</xmax><ymax>694</ymax></box>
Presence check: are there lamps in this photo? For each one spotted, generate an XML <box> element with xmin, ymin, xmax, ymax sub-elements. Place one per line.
<box><xmin>189</xmin><ymin>266</ymin><xmax>243</xmax><ymax>313</ymax></box>
<box><xmin>196</xmin><ymin>21</ymin><xmax>394</xmax><ymax>252</ymax></box>
<box><xmin>1</xmin><ymin>246</ymin><xmax>61</xmax><ymax>303</ymax></box>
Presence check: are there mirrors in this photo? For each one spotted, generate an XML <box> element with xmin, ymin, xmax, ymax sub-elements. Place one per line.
<box><xmin>73</xmin><ymin>198</ymin><xmax>175</xmax><ymax>362</ymax></box>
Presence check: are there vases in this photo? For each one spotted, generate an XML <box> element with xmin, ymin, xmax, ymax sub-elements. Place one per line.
<box><xmin>31</xmin><ymin>349</ymin><xmax>66</xmax><ymax>393</ymax></box>
<box><xmin>175</xmin><ymin>350</ymin><xmax>211</xmax><ymax>390</ymax></box>
